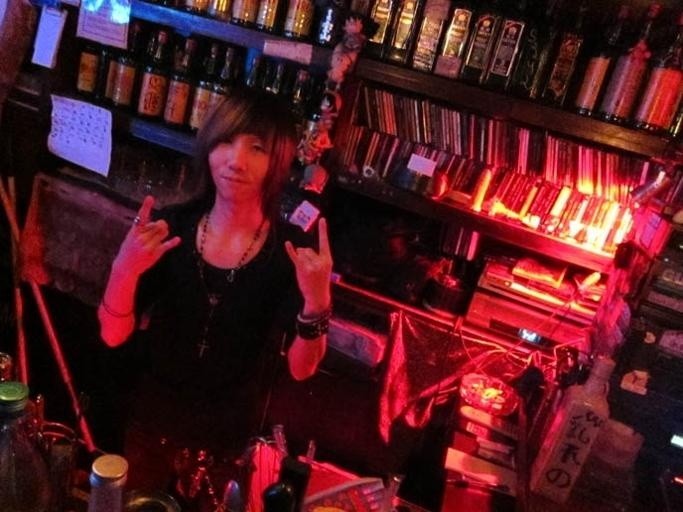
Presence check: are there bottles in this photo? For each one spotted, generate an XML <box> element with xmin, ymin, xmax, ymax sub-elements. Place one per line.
<box><xmin>516</xmin><ymin>352</ymin><xmax>618</xmax><ymax>512</ymax></box>
<box><xmin>70</xmin><ymin>0</ymin><xmax>683</xmax><ymax>168</ymax></box>
<box><xmin>0</xmin><ymin>352</ymin><xmax>130</xmax><ymax>512</ymax></box>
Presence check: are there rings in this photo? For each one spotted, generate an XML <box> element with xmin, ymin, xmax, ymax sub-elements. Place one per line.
<box><xmin>131</xmin><ymin>215</ymin><xmax>141</xmax><ymax>225</ymax></box>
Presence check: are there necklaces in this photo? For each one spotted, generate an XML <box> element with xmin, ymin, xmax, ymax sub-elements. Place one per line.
<box><xmin>194</xmin><ymin>210</ymin><xmax>266</xmax><ymax>308</ymax></box>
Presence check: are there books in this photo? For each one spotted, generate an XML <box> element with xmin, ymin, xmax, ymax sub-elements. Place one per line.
<box><xmin>342</xmin><ymin>76</ymin><xmax>667</xmax><ymax>280</ymax></box>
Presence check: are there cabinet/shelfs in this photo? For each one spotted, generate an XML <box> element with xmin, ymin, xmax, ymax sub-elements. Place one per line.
<box><xmin>0</xmin><ymin>0</ymin><xmax>682</xmax><ymax>512</ymax></box>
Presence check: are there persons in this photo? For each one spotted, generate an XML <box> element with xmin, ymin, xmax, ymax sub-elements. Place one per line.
<box><xmin>96</xmin><ymin>83</ymin><xmax>333</xmax><ymax>438</ymax></box>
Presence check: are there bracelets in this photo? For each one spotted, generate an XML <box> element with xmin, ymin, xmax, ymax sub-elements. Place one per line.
<box><xmin>99</xmin><ymin>296</ymin><xmax>134</xmax><ymax>320</ymax></box>
<box><xmin>292</xmin><ymin>308</ymin><xmax>330</xmax><ymax>339</ymax></box>
